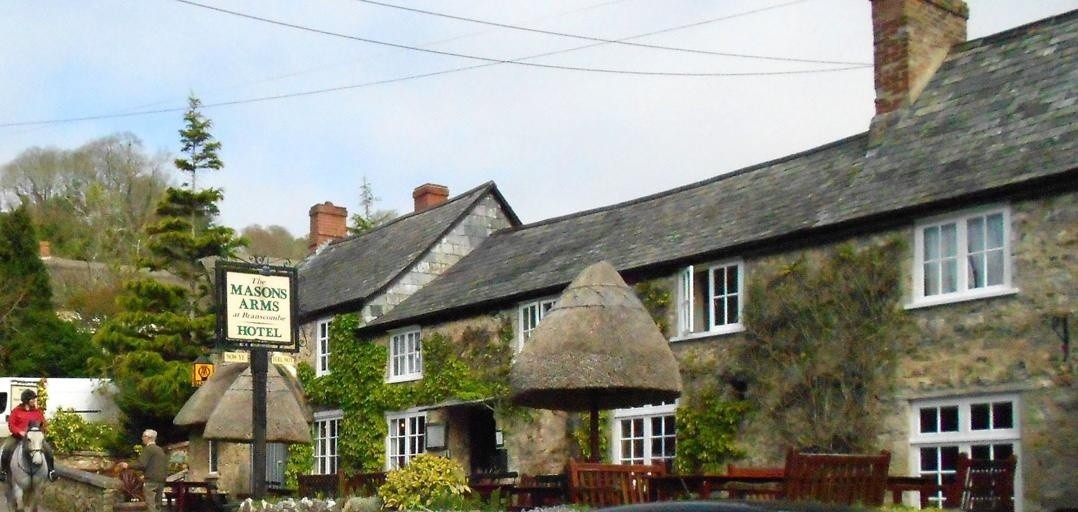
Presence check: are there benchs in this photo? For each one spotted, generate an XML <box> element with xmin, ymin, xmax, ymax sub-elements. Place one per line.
<box><xmin>163</xmin><ymin>480</ymin><xmax>237</xmax><ymax>512</ymax></box>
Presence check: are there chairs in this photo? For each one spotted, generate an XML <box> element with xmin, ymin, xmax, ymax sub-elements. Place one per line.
<box><xmin>297</xmin><ymin>469</ymin><xmax>565</xmax><ymax>512</ymax></box>
<box><xmin>726</xmin><ymin>448</ymin><xmax>889</xmax><ymax>505</ymax></box>
<box><xmin>944</xmin><ymin>449</ymin><xmax>1018</xmax><ymax>512</ymax></box>
<box><xmin>568</xmin><ymin>454</ymin><xmax>670</xmax><ymax>508</ymax></box>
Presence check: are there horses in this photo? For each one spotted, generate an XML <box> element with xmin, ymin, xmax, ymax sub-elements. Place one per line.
<box><xmin>0</xmin><ymin>418</ymin><xmax>49</xmax><ymax>512</ymax></box>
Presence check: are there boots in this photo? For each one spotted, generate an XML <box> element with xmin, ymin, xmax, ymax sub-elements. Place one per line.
<box><xmin>47</xmin><ymin>457</ymin><xmax>57</xmax><ymax>482</ymax></box>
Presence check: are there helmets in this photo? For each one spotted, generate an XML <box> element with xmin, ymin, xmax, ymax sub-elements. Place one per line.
<box><xmin>21</xmin><ymin>389</ymin><xmax>40</xmax><ymax>401</ymax></box>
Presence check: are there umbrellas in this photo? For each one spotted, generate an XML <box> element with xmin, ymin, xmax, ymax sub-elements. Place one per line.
<box><xmin>506</xmin><ymin>259</ymin><xmax>686</xmax><ymax>459</ymax></box>
<box><xmin>204</xmin><ymin>354</ymin><xmax>314</xmax><ymax>448</ymax></box>
<box><xmin>170</xmin><ymin>361</ymin><xmax>314</xmax><ymax>431</ymax></box>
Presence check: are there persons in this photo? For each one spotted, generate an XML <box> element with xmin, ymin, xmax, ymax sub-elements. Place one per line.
<box><xmin>116</xmin><ymin>426</ymin><xmax>169</xmax><ymax>511</ymax></box>
<box><xmin>0</xmin><ymin>388</ymin><xmax>59</xmax><ymax>483</ymax></box>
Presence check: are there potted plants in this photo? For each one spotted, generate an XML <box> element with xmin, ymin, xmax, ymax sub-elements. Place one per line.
<box><xmin>113</xmin><ymin>468</ymin><xmax>147</xmax><ymax>512</ymax></box>
